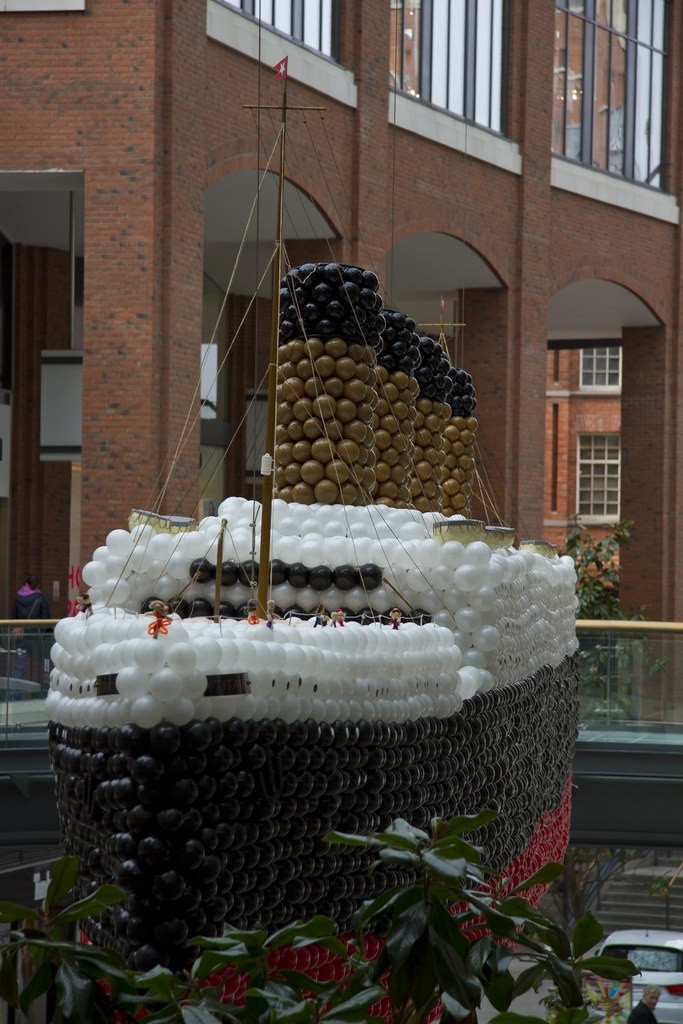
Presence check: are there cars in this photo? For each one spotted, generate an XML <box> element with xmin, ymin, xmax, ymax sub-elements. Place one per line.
<box><xmin>580</xmin><ymin>928</ymin><xmax>683</xmax><ymax>1024</ymax></box>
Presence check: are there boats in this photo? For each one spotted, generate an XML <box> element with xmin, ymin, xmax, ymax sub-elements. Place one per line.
<box><xmin>39</xmin><ymin>56</ymin><xmax>585</xmax><ymax>1024</ymax></box>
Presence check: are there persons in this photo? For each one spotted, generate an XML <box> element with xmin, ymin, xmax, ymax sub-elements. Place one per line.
<box><xmin>627</xmin><ymin>986</ymin><xmax>662</xmax><ymax>1024</ymax></box>
<box><xmin>580</xmin><ymin>559</ymin><xmax>621</xmax><ymax>700</ymax></box>
<box><xmin>9</xmin><ymin>575</ymin><xmax>51</xmax><ymax>701</ymax></box>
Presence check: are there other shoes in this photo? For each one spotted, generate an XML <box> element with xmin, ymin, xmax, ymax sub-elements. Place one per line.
<box><xmin>10</xmin><ymin>698</ymin><xmax>16</xmax><ymax>702</ymax></box>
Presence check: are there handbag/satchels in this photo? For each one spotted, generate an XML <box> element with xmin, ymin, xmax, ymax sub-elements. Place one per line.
<box><xmin>11</xmin><ymin>627</ymin><xmax>24</xmax><ymax>641</ymax></box>
